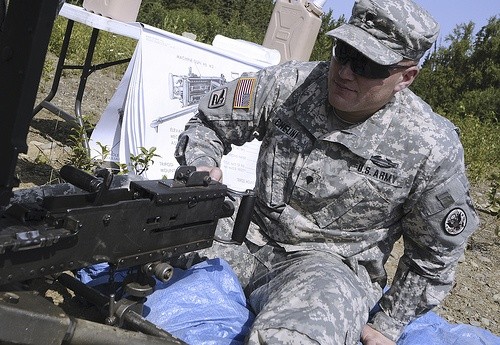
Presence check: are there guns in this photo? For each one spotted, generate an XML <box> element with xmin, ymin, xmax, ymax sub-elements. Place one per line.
<box><xmin>0</xmin><ymin>163</ymin><xmax>261</xmax><ymax>345</ymax></box>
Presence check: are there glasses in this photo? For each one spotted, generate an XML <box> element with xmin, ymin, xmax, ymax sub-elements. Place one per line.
<box><xmin>331</xmin><ymin>42</ymin><xmax>418</xmax><ymax>80</ymax></box>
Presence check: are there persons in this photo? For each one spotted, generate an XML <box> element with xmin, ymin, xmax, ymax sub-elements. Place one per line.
<box><xmin>0</xmin><ymin>0</ymin><xmax>480</xmax><ymax>345</ymax></box>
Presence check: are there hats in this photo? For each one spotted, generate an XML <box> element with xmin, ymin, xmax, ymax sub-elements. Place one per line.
<box><xmin>324</xmin><ymin>0</ymin><xmax>440</xmax><ymax>66</ymax></box>
